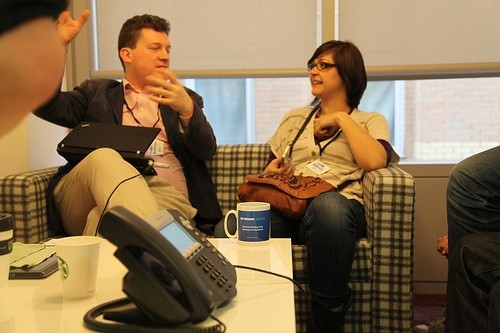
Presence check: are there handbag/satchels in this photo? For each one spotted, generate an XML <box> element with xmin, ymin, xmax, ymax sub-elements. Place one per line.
<box><xmin>238</xmin><ymin>173</ymin><xmax>336</xmax><ymax>220</ymax></box>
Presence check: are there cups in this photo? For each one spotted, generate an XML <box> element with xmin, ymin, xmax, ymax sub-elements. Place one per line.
<box><xmin>0</xmin><ymin>214</ymin><xmax>14</xmax><ymax>255</ymax></box>
<box><xmin>224</xmin><ymin>202</ymin><xmax>270</xmax><ymax>246</ymax></box>
<box><xmin>55</xmin><ymin>236</ymin><xmax>101</xmax><ymax>299</ymax></box>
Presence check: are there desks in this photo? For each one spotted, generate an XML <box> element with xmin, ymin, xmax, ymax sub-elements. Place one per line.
<box><xmin>0</xmin><ymin>235</ymin><xmax>296</xmax><ymax>333</ymax></box>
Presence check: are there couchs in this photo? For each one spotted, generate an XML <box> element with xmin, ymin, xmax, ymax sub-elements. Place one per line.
<box><xmin>0</xmin><ymin>144</ymin><xmax>415</xmax><ymax>333</ymax></box>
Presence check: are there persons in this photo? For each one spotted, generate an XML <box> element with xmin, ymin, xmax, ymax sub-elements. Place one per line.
<box><xmin>444</xmin><ymin>146</ymin><xmax>500</xmax><ymax>333</ymax></box>
<box><xmin>31</xmin><ymin>9</ymin><xmax>224</xmax><ymax>237</ymax></box>
<box><xmin>214</xmin><ymin>39</ymin><xmax>393</xmax><ymax>333</ymax></box>
<box><xmin>0</xmin><ymin>0</ymin><xmax>65</xmax><ymax>139</ymax></box>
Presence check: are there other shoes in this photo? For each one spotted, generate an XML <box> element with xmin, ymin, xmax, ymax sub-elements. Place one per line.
<box><xmin>412</xmin><ymin>316</ymin><xmax>445</xmax><ymax>333</ymax></box>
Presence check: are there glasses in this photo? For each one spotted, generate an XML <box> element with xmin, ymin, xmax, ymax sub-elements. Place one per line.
<box><xmin>308</xmin><ymin>62</ymin><xmax>337</xmax><ymax>71</ymax></box>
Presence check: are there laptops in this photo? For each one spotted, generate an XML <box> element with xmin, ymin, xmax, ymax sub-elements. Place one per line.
<box><xmin>56</xmin><ymin>121</ymin><xmax>161</xmax><ymax>177</ymax></box>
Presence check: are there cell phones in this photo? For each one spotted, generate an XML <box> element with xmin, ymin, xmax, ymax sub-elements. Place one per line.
<box><xmin>282</xmin><ymin>146</ymin><xmax>291</xmax><ymax>166</ymax></box>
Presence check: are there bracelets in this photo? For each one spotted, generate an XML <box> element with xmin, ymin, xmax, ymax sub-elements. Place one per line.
<box><xmin>179</xmin><ymin>114</ymin><xmax>193</xmax><ymax>119</ymax></box>
